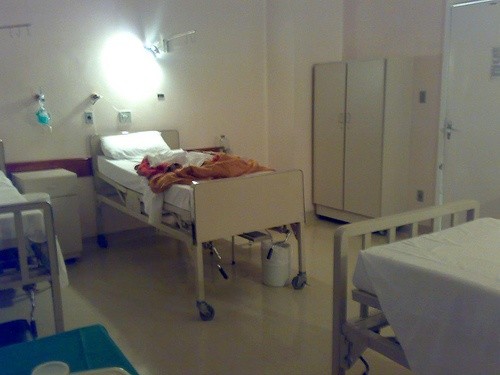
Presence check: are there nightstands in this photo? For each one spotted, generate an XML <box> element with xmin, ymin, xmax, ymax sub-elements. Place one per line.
<box><xmin>11</xmin><ymin>169</ymin><xmax>84</xmax><ymax>264</ymax></box>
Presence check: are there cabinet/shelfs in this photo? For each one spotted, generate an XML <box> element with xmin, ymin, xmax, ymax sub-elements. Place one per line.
<box><xmin>311</xmin><ymin>57</ymin><xmax>414</xmax><ymax>235</ymax></box>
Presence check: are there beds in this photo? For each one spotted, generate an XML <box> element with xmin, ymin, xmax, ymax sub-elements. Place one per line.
<box><xmin>332</xmin><ymin>199</ymin><xmax>500</xmax><ymax>374</ymax></box>
<box><xmin>0</xmin><ymin>142</ymin><xmax>66</xmax><ymax>335</ymax></box>
<box><xmin>89</xmin><ymin>130</ymin><xmax>307</xmax><ymax>322</ymax></box>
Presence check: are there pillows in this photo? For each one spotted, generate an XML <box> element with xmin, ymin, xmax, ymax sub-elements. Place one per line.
<box><xmin>99</xmin><ymin>130</ymin><xmax>170</xmax><ymax>161</ymax></box>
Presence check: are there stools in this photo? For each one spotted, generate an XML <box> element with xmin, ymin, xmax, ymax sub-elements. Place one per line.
<box><xmin>232</xmin><ymin>231</ymin><xmax>273</xmax><ymax>265</ymax></box>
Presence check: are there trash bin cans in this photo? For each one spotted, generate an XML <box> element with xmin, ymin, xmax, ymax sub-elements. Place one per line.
<box><xmin>261</xmin><ymin>241</ymin><xmax>291</xmax><ymax>287</ymax></box>
